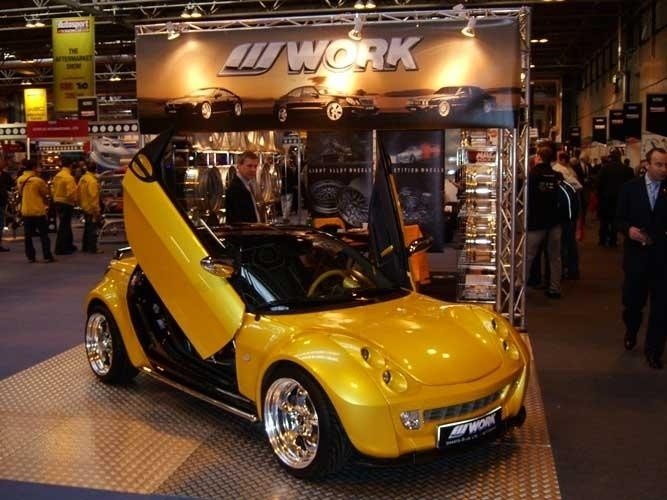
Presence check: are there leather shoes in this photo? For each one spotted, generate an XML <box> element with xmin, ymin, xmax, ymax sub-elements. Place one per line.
<box><xmin>56</xmin><ymin>250</ymin><xmax>73</xmax><ymax>255</ymax></box>
<box><xmin>0</xmin><ymin>246</ymin><xmax>11</xmax><ymax>252</ymax></box>
<box><xmin>545</xmin><ymin>290</ymin><xmax>560</xmax><ymax>300</ymax></box>
<box><xmin>69</xmin><ymin>245</ymin><xmax>77</xmax><ymax>250</ymax></box>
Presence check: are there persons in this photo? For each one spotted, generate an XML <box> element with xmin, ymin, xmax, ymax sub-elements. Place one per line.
<box><xmin>223</xmin><ymin>151</ymin><xmax>262</xmax><ymax>224</ymax></box>
<box><xmin>277</xmin><ymin>157</ymin><xmax>296</xmax><ymax>222</ymax></box>
<box><xmin>77</xmin><ymin>158</ymin><xmax>105</xmax><ymax>254</ymax></box>
<box><xmin>596</xmin><ymin>147</ymin><xmax>620</xmax><ymax>250</ymax></box>
<box><xmin>0</xmin><ymin>156</ymin><xmax>17</xmax><ymax>252</ymax></box>
<box><xmin>16</xmin><ymin>159</ymin><xmax>57</xmax><ymax>264</ymax></box>
<box><xmin>51</xmin><ymin>155</ymin><xmax>80</xmax><ymax>256</ymax></box>
<box><xmin>525</xmin><ymin>146</ymin><xmax>562</xmax><ymax>298</ymax></box>
<box><xmin>538</xmin><ymin>146</ymin><xmax>583</xmax><ymax>280</ymax></box>
<box><xmin>612</xmin><ymin>147</ymin><xmax>667</xmax><ymax>369</ymax></box>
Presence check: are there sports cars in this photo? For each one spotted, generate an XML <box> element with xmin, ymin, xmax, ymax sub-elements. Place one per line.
<box><xmin>84</xmin><ymin>129</ymin><xmax>531</xmax><ymax>481</ymax></box>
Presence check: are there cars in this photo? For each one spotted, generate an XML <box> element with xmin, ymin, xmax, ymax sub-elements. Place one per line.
<box><xmin>165</xmin><ymin>87</ymin><xmax>242</xmax><ymax>120</ymax></box>
<box><xmin>272</xmin><ymin>85</ymin><xmax>379</xmax><ymax>122</ymax></box>
<box><xmin>396</xmin><ymin>144</ymin><xmax>439</xmax><ymax>163</ymax></box>
<box><xmin>406</xmin><ymin>86</ymin><xmax>496</xmax><ymax>118</ymax></box>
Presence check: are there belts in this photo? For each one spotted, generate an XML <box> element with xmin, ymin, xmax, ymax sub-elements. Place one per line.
<box><xmin>624</xmin><ymin>329</ymin><xmax>637</xmax><ymax>351</ymax></box>
<box><xmin>645</xmin><ymin>351</ymin><xmax>664</xmax><ymax>373</ymax></box>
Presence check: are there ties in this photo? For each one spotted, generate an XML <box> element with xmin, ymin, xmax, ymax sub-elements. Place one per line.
<box><xmin>649</xmin><ymin>183</ymin><xmax>657</xmax><ymax>212</ymax></box>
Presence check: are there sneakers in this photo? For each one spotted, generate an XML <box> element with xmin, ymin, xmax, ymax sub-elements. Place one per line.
<box><xmin>92</xmin><ymin>249</ymin><xmax>104</xmax><ymax>254</ymax></box>
<box><xmin>44</xmin><ymin>256</ymin><xmax>54</xmax><ymax>261</ymax></box>
<box><xmin>28</xmin><ymin>257</ymin><xmax>35</xmax><ymax>262</ymax></box>
